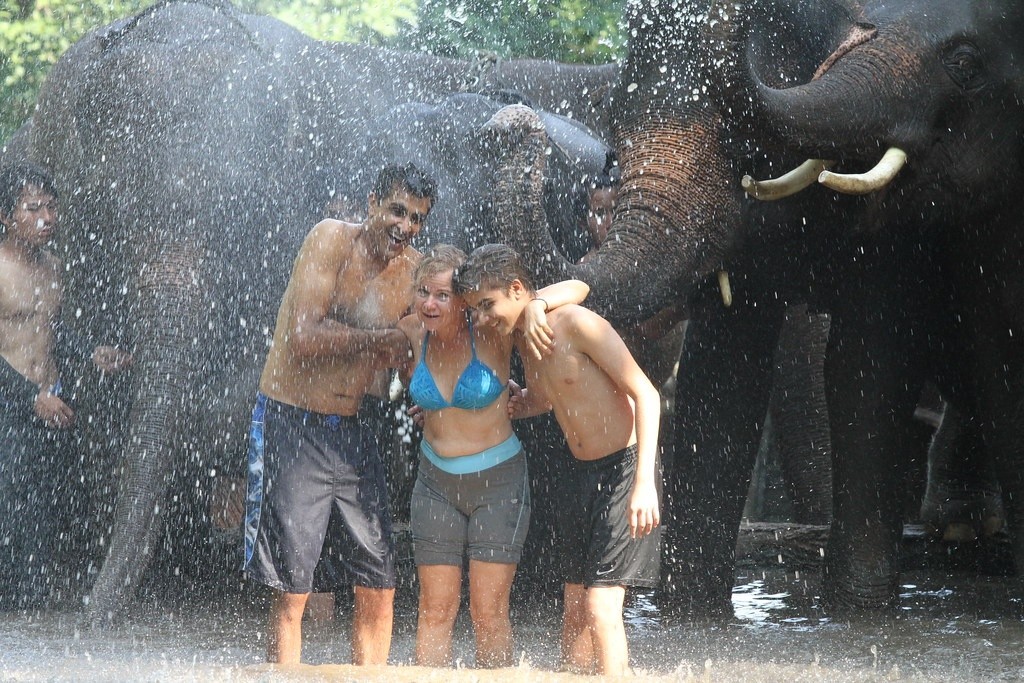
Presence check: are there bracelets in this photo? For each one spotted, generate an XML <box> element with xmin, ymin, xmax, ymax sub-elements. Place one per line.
<box><xmin>529</xmin><ymin>298</ymin><xmax>549</xmax><ymax>312</ymax></box>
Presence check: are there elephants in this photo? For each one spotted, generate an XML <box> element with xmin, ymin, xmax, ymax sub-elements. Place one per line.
<box><xmin>1</xmin><ymin>0</ymin><xmax>1024</xmax><ymax>630</ymax></box>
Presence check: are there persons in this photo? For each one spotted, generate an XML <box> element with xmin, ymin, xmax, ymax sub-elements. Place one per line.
<box><xmin>0</xmin><ymin>163</ymin><xmax>137</xmax><ymax>617</ymax></box>
<box><xmin>241</xmin><ymin>163</ymin><xmax>664</xmax><ymax>678</ymax></box>
